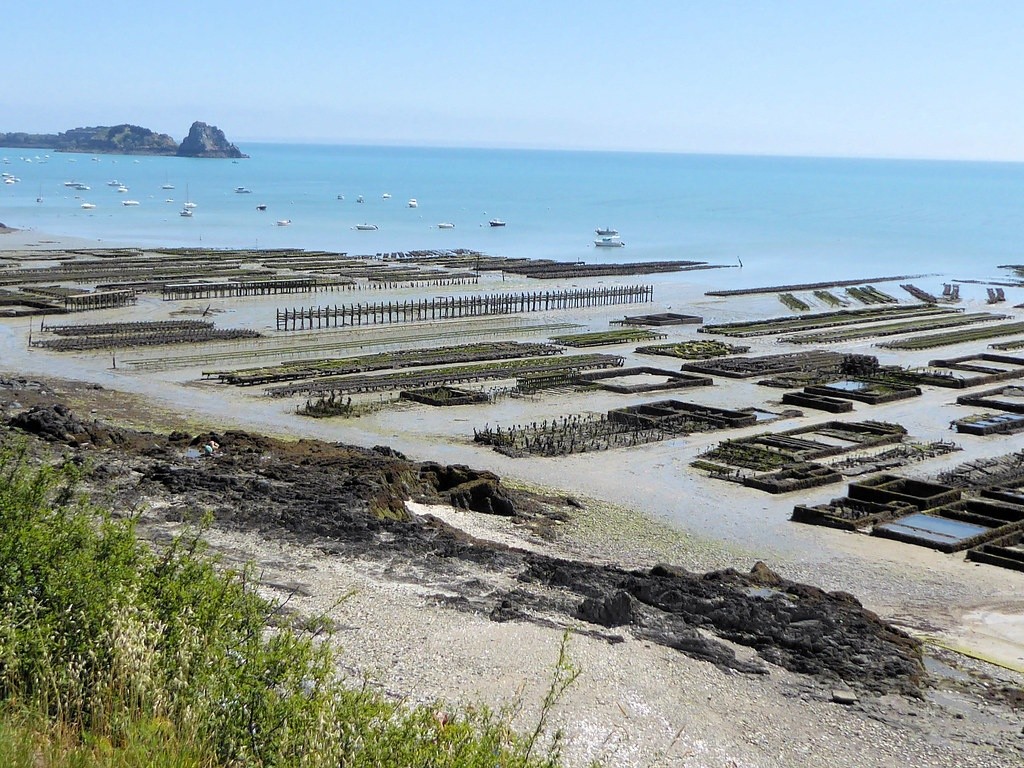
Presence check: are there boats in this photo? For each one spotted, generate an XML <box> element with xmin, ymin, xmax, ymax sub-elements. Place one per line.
<box><xmin>595</xmin><ymin>226</ymin><xmax>619</xmax><ymax>237</ymax></box>
<box><xmin>593</xmin><ymin>236</ymin><xmax>626</xmax><ymax>247</ymax></box>
<box><xmin>184</xmin><ymin>202</ymin><xmax>198</xmax><ymax>209</ymax></box>
<box><xmin>81</xmin><ymin>203</ymin><xmax>97</xmax><ymax>209</ymax></box>
<box><xmin>489</xmin><ymin>217</ymin><xmax>506</xmax><ymax>227</ymax></box>
<box><xmin>180</xmin><ymin>208</ymin><xmax>194</xmax><ymax>217</ymax></box>
<box><xmin>437</xmin><ymin>223</ymin><xmax>454</xmax><ymax>229</ymax></box>
<box><xmin>356</xmin><ymin>224</ymin><xmax>379</xmax><ymax>231</ymax></box>
<box><xmin>277</xmin><ymin>219</ymin><xmax>292</xmax><ymax>227</ymax></box>
